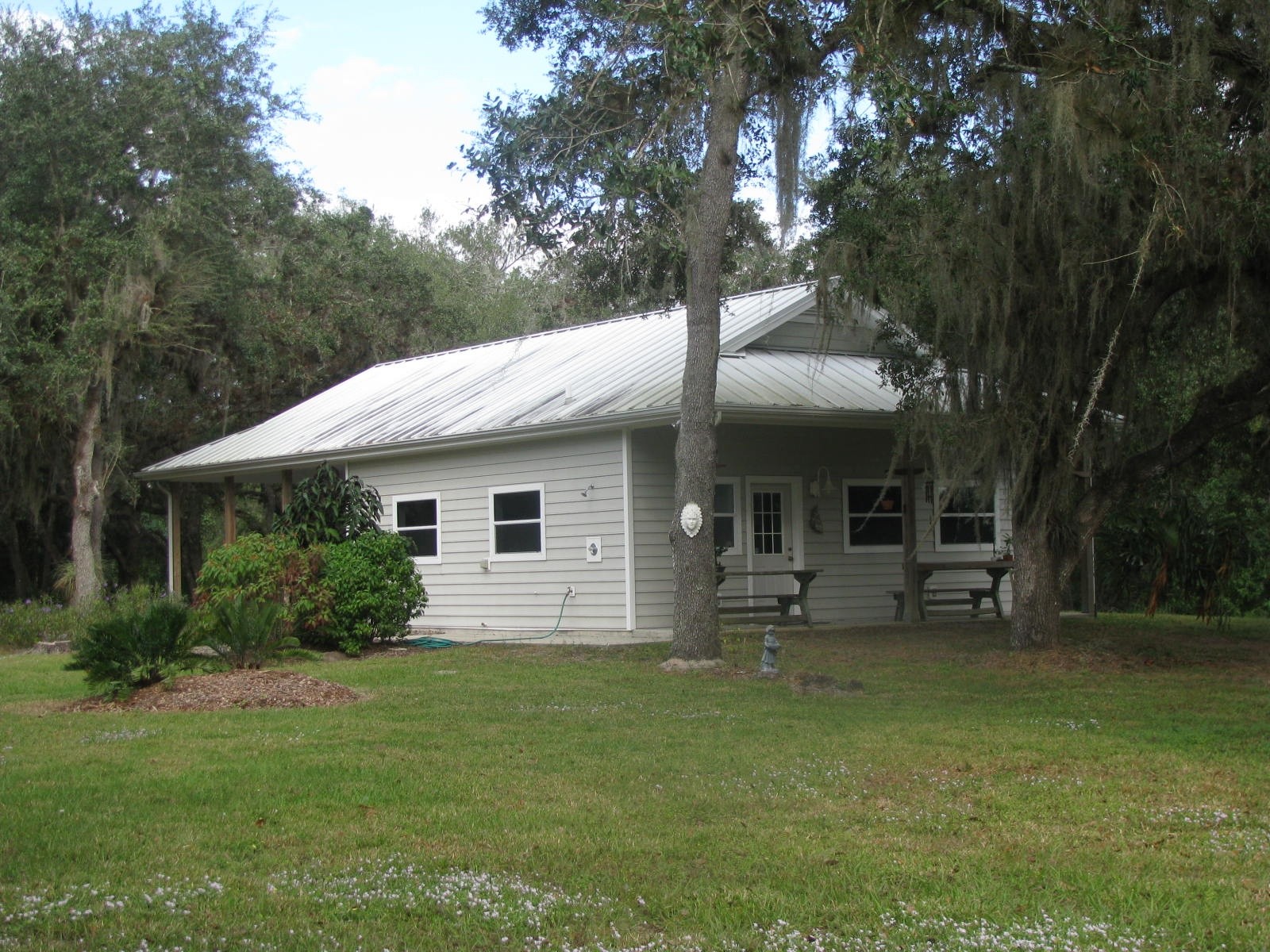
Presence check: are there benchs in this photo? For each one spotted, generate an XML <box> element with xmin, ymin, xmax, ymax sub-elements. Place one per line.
<box><xmin>715</xmin><ymin>568</ymin><xmax>823</xmax><ymax>627</ymax></box>
<box><xmin>886</xmin><ymin>560</ymin><xmax>1014</xmax><ymax>623</ymax></box>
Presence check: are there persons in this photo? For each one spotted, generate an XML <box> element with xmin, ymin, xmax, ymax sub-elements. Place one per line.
<box><xmin>685</xmin><ymin>511</ymin><xmax>699</xmax><ymax>529</ymax></box>
<box><xmin>761</xmin><ymin>624</ymin><xmax>783</xmax><ymax>669</ymax></box>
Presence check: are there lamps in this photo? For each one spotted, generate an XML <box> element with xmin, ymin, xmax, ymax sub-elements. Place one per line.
<box><xmin>580</xmin><ymin>484</ymin><xmax>594</xmax><ymax>497</ymax></box>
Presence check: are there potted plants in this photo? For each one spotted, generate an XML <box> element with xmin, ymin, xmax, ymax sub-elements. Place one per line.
<box><xmin>1001</xmin><ymin>533</ymin><xmax>1013</xmax><ymax>560</ymax></box>
